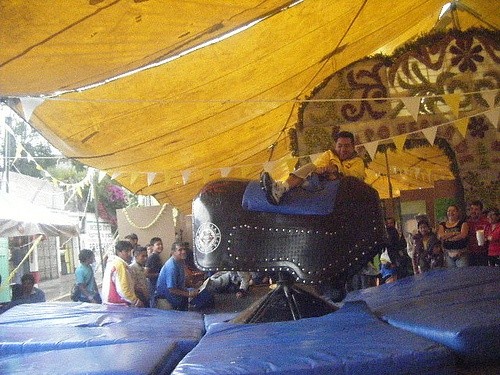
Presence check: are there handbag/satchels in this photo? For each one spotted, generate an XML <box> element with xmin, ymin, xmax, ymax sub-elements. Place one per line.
<box><xmin>70</xmin><ymin>283</ymin><xmax>82</xmax><ymax>302</ymax></box>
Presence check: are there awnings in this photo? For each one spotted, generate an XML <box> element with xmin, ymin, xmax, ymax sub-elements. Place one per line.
<box><xmin>0</xmin><ymin>191</ymin><xmax>80</xmax><ymax>239</ymax></box>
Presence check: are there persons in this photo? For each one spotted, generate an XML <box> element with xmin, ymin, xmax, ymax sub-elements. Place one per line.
<box><xmin>102</xmin><ymin>240</ymin><xmax>146</xmax><ymax>307</ymax></box>
<box><xmin>70</xmin><ymin>249</ymin><xmax>102</xmax><ymax>304</ymax></box>
<box><xmin>124</xmin><ymin>231</ymin><xmax>278</xmax><ymax>312</ymax></box>
<box><xmin>0</xmin><ymin>271</ymin><xmax>46</xmax><ymax>313</ymax></box>
<box><xmin>261</xmin><ymin>131</ymin><xmax>366</xmax><ymax>206</ymax></box>
<box><xmin>345</xmin><ymin>199</ymin><xmax>500</xmax><ymax>296</ymax></box>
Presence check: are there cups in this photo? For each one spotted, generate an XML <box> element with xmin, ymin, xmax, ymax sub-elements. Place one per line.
<box><xmin>476</xmin><ymin>230</ymin><xmax>484</xmax><ymax>246</ymax></box>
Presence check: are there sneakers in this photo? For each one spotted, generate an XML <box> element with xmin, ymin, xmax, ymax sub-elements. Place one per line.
<box><xmin>261</xmin><ymin>171</ymin><xmax>287</xmax><ymax>206</ymax></box>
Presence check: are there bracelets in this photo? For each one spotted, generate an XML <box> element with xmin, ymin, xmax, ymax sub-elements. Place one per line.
<box><xmin>453</xmin><ymin>232</ymin><xmax>457</xmax><ymax>236</ymax></box>
